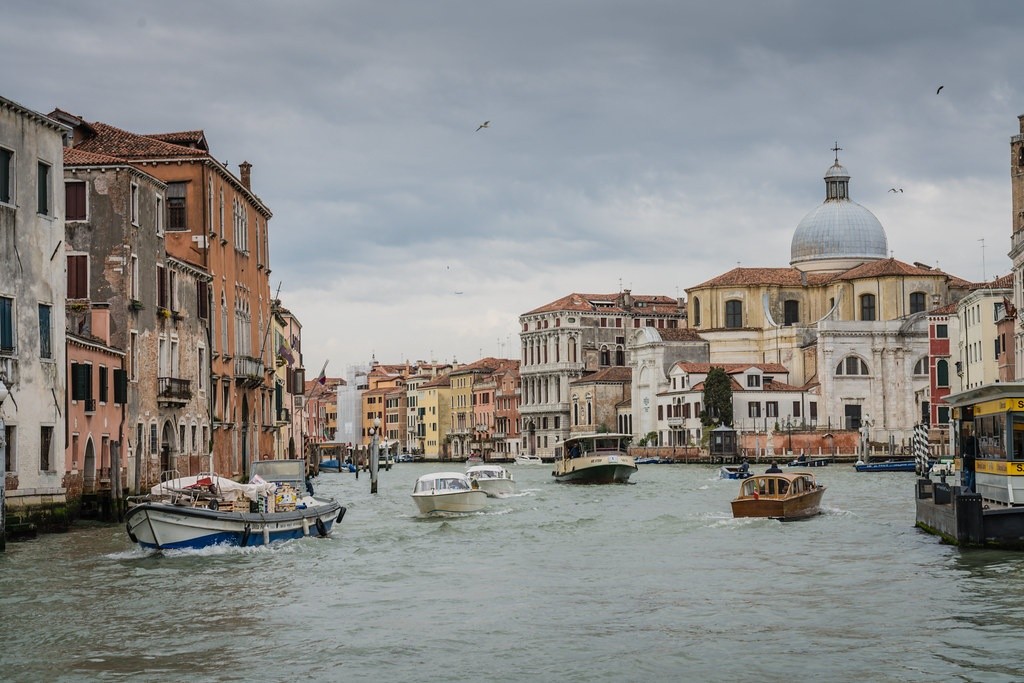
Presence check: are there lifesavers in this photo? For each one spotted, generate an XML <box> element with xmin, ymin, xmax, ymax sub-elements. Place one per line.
<box><xmin>210</xmin><ymin>499</ymin><xmax>219</xmax><ymax>510</ymax></box>
<box><xmin>125</xmin><ymin>523</ymin><xmax>138</xmax><ymax>543</ymax></box>
<box><xmin>242</xmin><ymin>527</ymin><xmax>251</xmax><ymax>546</ymax></box>
<box><xmin>316</xmin><ymin>518</ymin><xmax>327</xmax><ymax>536</ymax></box>
<box><xmin>336</xmin><ymin>507</ymin><xmax>346</xmax><ymax>523</ymax></box>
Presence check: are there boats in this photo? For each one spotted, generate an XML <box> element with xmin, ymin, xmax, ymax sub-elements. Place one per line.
<box><xmin>721</xmin><ymin>467</ymin><xmax>753</xmax><ymax>479</ymax></box>
<box><xmin>552</xmin><ymin>433</ymin><xmax>638</xmax><ymax>485</ymax></box>
<box><xmin>318</xmin><ymin>455</ymin><xmax>394</xmax><ymax>473</ymax></box>
<box><xmin>853</xmin><ymin>460</ymin><xmax>942</xmax><ymax>471</ymax></box>
<box><xmin>730</xmin><ymin>471</ymin><xmax>827</xmax><ymax>522</ymax></box>
<box><xmin>410</xmin><ymin>454</ymin><xmax>543</xmax><ymax>519</ymax></box>
<box><xmin>787</xmin><ymin>458</ymin><xmax>829</xmax><ymax>466</ymax></box>
<box><xmin>125</xmin><ymin>471</ymin><xmax>348</xmax><ymax>555</ymax></box>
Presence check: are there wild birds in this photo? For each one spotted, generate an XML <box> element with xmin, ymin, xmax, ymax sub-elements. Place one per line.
<box><xmin>476</xmin><ymin>121</ymin><xmax>490</xmax><ymax>131</ymax></box>
<box><xmin>888</xmin><ymin>188</ymin><xmax>903</xmax><ymax>193</ymax></box>
<box><xmin>937</xmin><ymin>85</ymin><xmax>944</xmax><ymax>95</ymax></box>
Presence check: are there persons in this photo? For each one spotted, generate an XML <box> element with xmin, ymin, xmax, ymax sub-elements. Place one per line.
<box><xmin>741</xmin><ymin>458</ymin><xmax>749</xmax><ymax>472</ymax></box>
<box><xmin>798</xmin><ymin>454</ymin><xmax>806</xmax><ymax>462</ymax></box>
<box><xmin>766</xmin><ymin>461</ymin><xmax>785</xmax><ymax>493</ymax></box>
<box><xmin>966</xmin><ymin>429</ymin><xmax>982</xmax><ymax>489</ymax></box>
<box><xmin>305</xmin><ymin>474</ymin><xmax>314</xmax><ymax>496</ymax></box>
<box><xmin>570</xmin><ymin>443</ymin><xmax>580</xmax><ymax>458</ymax></box>
